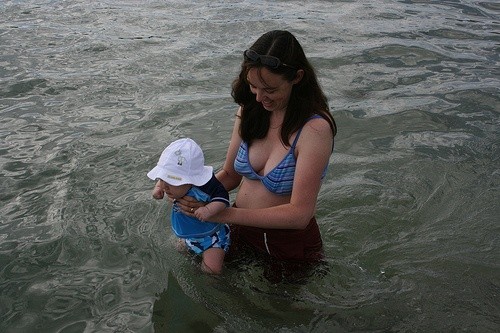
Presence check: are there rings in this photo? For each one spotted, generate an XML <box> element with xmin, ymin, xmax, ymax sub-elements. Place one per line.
<box><xmin>191</xmin><ymin>208</ymin><xmax>194</xmax><ymax>213</ymax></box>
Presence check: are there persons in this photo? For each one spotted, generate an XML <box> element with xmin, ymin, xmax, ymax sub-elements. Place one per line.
<box><xmin>147</xmin><ymin>138</ymin><xmax>229</xmax><ymax>275</ymax></box>
<box><xmin>176</xmin><ymin>29</ymin><xmax>337</xmax><ymax>268</ymax></box>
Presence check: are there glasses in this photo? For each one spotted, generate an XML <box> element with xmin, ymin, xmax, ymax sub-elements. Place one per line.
<box><xmin>245</xmin><ymin>50</ymin><xmax>291</xmax><ymax>72</ymax></box>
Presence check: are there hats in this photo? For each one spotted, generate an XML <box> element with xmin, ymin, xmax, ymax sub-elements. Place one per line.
<box><xmin>147</xmin><ymin>138</ymin><xmax>213</xmax><ymax>186</ymax></box>
